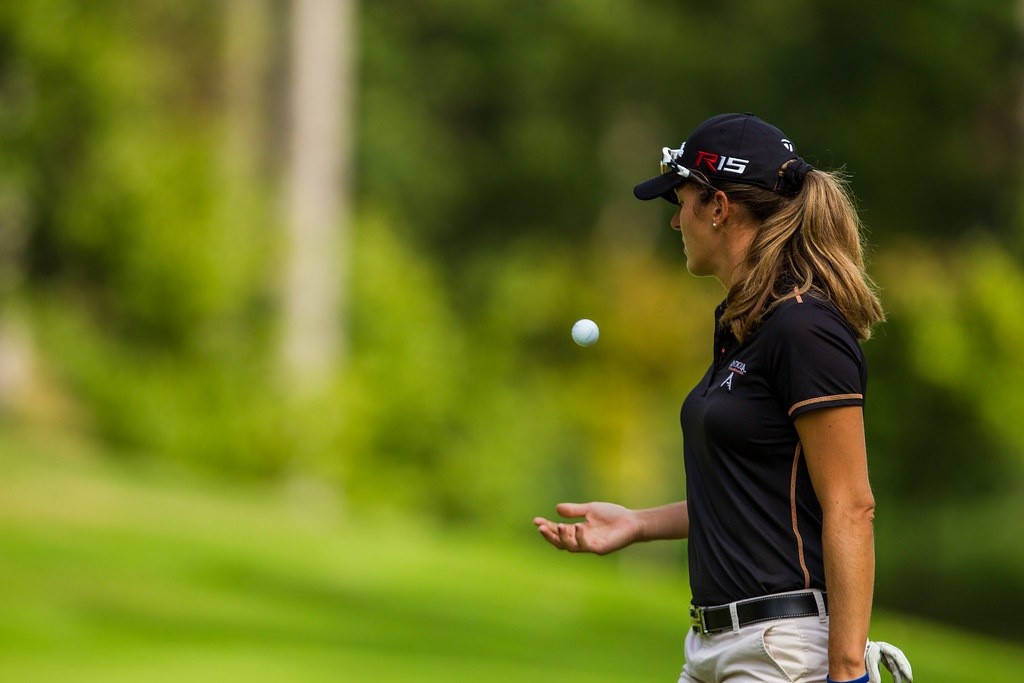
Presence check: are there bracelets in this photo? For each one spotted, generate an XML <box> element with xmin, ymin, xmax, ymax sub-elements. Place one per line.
<box><xmin>827</xmin><ymin>671</ymin><xmax>869</xmax><ymax>683</ymax></box>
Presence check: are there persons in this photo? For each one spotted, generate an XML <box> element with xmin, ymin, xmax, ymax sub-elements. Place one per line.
<box><xmin>533</xmin><ymin>111</ymin><xmax>888</xmax><ymax>683</ymax></box>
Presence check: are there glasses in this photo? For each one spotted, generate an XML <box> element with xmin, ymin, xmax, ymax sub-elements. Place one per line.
<box><xmin>660</xmin><ymin>146</ymin><xmax>717</xmax><ymax>192</ymax></box>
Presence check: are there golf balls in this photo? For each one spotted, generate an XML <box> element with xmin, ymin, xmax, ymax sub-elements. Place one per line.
<box><xmin>571</xmin><ymin>319</ymin><xmax>601</xmax><ymax>349</ymax></box>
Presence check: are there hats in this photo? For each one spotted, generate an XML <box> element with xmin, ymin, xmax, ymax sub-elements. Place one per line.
<box><xmin>634</xmin><ymin>112</ymin><xmax>801</xmax><ymax>208</ymax></box>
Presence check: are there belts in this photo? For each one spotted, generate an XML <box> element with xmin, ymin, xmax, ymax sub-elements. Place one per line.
<box><xmin>690</xmin><ymin>592</ymin><xmax>829</xmax><ymax>635</ymax></box>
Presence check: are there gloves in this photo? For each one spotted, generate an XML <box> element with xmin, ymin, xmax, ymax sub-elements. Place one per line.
<box><xmin>867</xmin><ymin>640</ymin><xmax>913</xmax><ymax>683</ymax></box>
